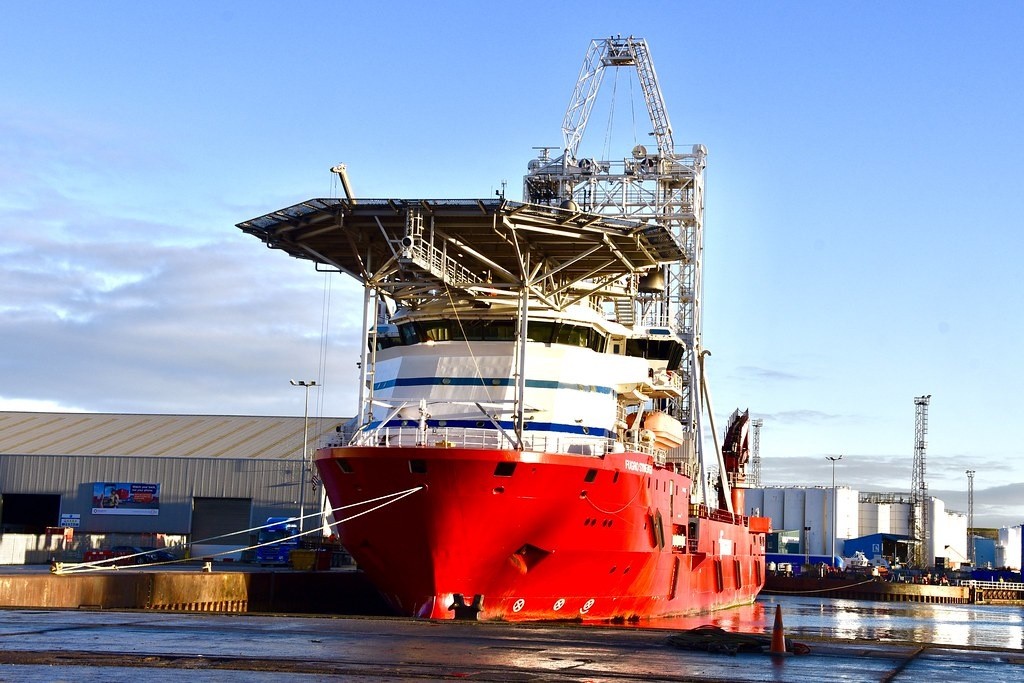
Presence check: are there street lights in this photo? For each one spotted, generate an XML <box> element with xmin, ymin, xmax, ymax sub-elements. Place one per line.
<box><xmin>289</xmin><ymin>378</ymin><xmax>318</xmax><ymax>538</ymax></box>
<box><xmin>825</xmin><ymin>454</ymin><xmax>843</xmax><ymax>568</ymax></box>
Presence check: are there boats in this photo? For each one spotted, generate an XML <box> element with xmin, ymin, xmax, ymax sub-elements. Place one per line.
<box><xmin>233</xmin><ymin>31</ymin><xmax>773</xmax><ymax>626</ymax></box>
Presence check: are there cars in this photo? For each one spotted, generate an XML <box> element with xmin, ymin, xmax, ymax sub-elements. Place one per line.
<box><xmin>111</xmin><ymin>544</ymin><xmax>179</xmax><ymax>564</ymax></box>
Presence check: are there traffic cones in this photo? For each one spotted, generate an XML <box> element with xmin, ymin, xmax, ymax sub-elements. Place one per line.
<box><xmin>762</xmin><ymin>604</ymin><xmax>795</xmax><ymax>657</ymax></box>
